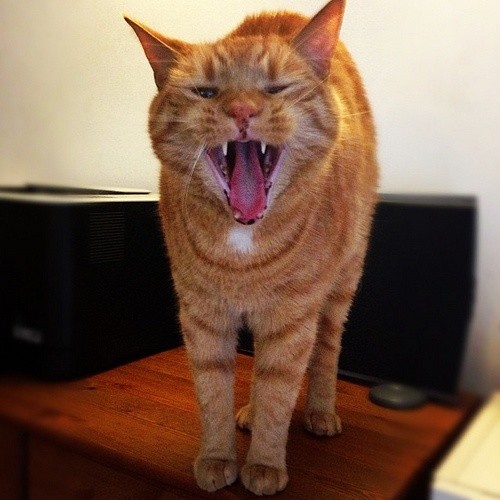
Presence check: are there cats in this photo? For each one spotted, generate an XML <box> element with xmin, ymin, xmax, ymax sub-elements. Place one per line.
<box><xmin>122</xmin><ymin>0</ymin><xmax>379</xmax><ymax>495</ymax></box>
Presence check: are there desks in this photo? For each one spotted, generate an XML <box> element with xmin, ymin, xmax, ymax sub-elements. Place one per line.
<box><xmin>0</xmin><ymin>343</ymin><xmax>481</xmax><ymax>500</ymax></box>
